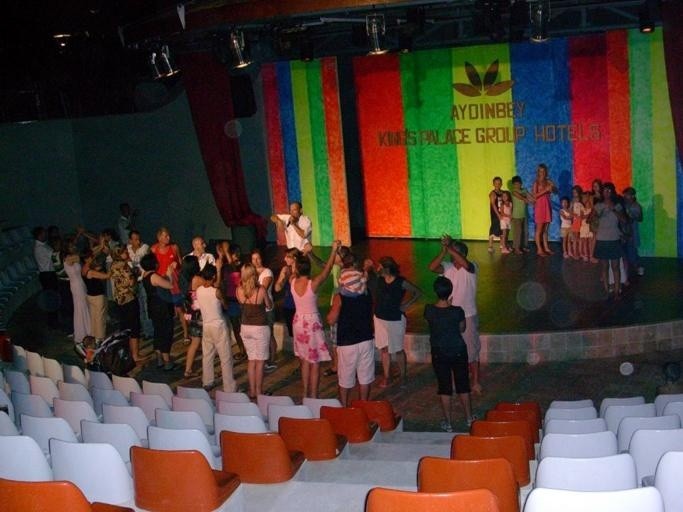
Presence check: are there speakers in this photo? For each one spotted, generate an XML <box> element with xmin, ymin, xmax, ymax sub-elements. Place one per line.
<box><xmin>229</xmin><ymin>73</ymin><xmax>258</xmax><ymax>118</ymax></box>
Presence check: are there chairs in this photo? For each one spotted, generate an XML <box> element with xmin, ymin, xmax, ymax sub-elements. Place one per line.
<box><xmin>546</xmin><ymin>418</ymin><xmax>608</xmax><ymax>434</ymax></box>
<box><xmin>486</xmin><ymin>402</ymin><xmax>542</xmax><ymax>444</ymax></box>
<box><xmin>1</xmin><ymin>478</ymin><xmax>134</xmax><ymax>512</ymax></box>
<box><xmin>278</xmin><ymin>416</ymin><xmax>350</xmax><ymax>461</ymax></box>
<box><xmin>539</xmin><ymin>432</ymin><xmax>618</xmax><ymax>460</ymax></box>
<box><xmin>364</xmin><ymin>487</ymin><xmax>499</xmax><ymax>512</ymax></box>
<box><xmin>219</xmin><ymin>430</ymin><xmax>308</xmax><ymax>484</ymax></box>
<box><xmin>600</xmin><ymin>395</ymin><xmax>683</xmax><ymax>452</ymax></box>
<box><xmin>545</xmin><ymin>398</ymin><xmax>600</xmax><ymax>418</ymax></box>
<box><xmin>449</xmin><ymin>434</ymin><xmax>531</xmax><ymax>487</ymax></box>
<box><xmin>130</xmin><ymin>445</ymin><xmax>242</xmax><ymax>512</ymax></box>
<box><xmin>146</xmin><ymin>426</ymin><xmax>215</xmax><ymax>470</ymax></box>
<box><xmin>213</xmin><ymin>414</ymin><xmax>268</xmax><ymax>445</ymax></box>
<box><xmin>534</xmin><ymin>454</ymin><xmax>638</xmax><ymax>490</ymax></box>
<box><xmin>80</xmin><ymin>418</ymin><xmax>141</xmax><ymax>464</ymax></box>
<box><xmin>522</xmin><ymin>487</ymin><xmax>664</xmax><ymax>512</ymax></box>
<box><xmin>0</xmin><ymin>436</ymin><xmax>54</xmax><ymax>481</ymax></box>
<box><xmin>319</xmin><ymin>406</ymin><xmax>381</xmax><ymax>443</ymax></box>
<box><xmin>352</xmin><ymin>399</ymin><xmax>403</xmax><ymax>431</ymax></box>
<box><xmin>621</xmin><ymin>428</ymin><xmax>683</xmax><ymax>485</ymax></box>
<box><xmin>470</xmin><ymin>420</ymin><xmax>535</xmax><ymax>462</ymax></box>
<box><xmin>416</xmin><ymin>456</ymin><xmax>520</xmax><ymax>512</ymax></box>
<box><xmin>19</xmin><ymin>413</ymin><xmax>79</xmax><ymax>462</ymax></box>
<box><xmin>1</xmin><ymin>410</ymin><xmax>21</xmax><ymax>435</ymax></box>
<box><xmin>48</xmin><ymin>438</ymin><xmax>133</xmax><ymax>504</ymax></box>
<box><xmin>0</xmin><ymin>345</ymin><xmax>344</xmax><ymax>433</ymax></box>
<box><xmin>643</xmin><ymin>452</ymin><xmax>683</xmax><ymax>511</ymax></box>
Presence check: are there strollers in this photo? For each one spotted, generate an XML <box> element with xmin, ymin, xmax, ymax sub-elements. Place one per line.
<box><xmin>73</xmin><ymin>326</ymin><xmax>136</xmax><ymax>383</ymax></box>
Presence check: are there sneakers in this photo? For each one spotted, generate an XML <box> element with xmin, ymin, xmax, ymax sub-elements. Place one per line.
<box><xmin>466</xmin><ymin>415</ymin><xmax>478</xmax><ymax>426</ymax></box>
<box><xmin>602</xmin><ymin>266</ymin><xmax>644</xmax><ymax>301</ymax></box>
<box><xmin>440</xmin><ymin>421</ymin><xmax>455</xmax><ymax>433</ymax></box>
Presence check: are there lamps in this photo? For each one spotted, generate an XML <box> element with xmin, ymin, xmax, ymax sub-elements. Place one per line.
<box><xmin>361</xmin><ymin>1</ymin><xmax>391</xmax><ymax>58</ymax></box>
<box><xmin>143</xmin><ymin>22</ymin><xmax>253</xmax><ymax>80</ymax></box>
<box><xmin>637</xmin><ymin>0</ymin><xmax>658</xmax><ymax>33</ymax></box>
<box><xmin>525</xmin><ymin>1</ymin><xmax>552</xmax><ymax>42</ymax></box>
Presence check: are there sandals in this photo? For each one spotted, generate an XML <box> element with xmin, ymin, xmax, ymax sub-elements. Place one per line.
<box><xmin>135</xmin><ymin>335</ymin><xmax>410</xmax><ymax>399</ymax></box>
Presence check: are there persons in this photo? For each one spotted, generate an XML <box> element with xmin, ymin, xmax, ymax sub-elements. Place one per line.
<box><xmin>488</xmin><ymin>164</ymin><xmax>644</xmax><ymax>302</ymax></box>
<box><xmin>424</xmin><ymin>276</ymin><xmax>480</xmax><ymax>431</ymax></box>
<box><xmin>655</xmin><ymin>361</ymin><xmax>682</xmax><ymax>397</ymax></box>
<box><xmin>428</xmin><ymin>232</ymin><xmax>483</xmax><ymax>396</ymax></box>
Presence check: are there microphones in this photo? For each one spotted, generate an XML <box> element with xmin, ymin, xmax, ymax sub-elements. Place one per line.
<box><xmin>286</xmin><ymin>221</ymin><xmax>291</xmax><ymax>227</ymax></box>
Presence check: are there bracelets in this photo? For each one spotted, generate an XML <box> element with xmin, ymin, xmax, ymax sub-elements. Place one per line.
<box><xmin>441</xmin><ymin>246</ymin><xmax>447</xmax><ymax>251</ymax></box>
